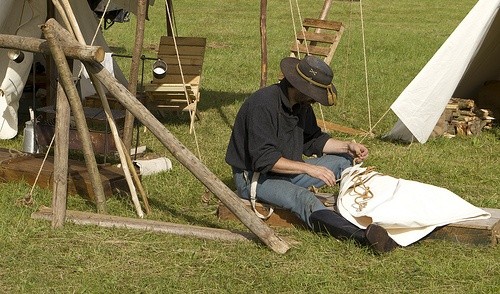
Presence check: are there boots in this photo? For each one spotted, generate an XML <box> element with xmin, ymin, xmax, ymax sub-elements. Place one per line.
<box><xmin>309</xmin><ymin>210</ymin><xmax>399</xmax><ymax>254</ymax></box>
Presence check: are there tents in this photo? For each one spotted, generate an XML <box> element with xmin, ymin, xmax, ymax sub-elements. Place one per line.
<box><xmin>0</xmin><ymin>0</ymin><xmax>155</xmax><ymax>144</ymax></box>
<box><xmin>379</xmin><ymin>0</ymin><xmax>500</xmax><ymax>145</ymax></box>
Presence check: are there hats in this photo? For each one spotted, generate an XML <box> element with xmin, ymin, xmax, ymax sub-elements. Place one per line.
<box><xmin>280</xmin><ymin>55</ymin><xmax>339</xmax><ymax>106</ymax></box>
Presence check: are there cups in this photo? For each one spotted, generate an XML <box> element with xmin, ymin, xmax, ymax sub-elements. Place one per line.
<box><xmin>151</xmin><ymin>58</ymin><xmax>168</xmax><ymax>80</ymax></box>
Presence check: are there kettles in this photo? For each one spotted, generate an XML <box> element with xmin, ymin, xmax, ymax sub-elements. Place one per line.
<box><xmin>23</xmin><ymin>119</ymin><xmax>40</xmax><ymax>154</ymax></box>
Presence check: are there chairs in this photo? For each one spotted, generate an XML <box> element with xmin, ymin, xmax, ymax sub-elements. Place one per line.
<box><xmin>143</xmin><ymin>34</ymin><xmax>208</xmax><ymax>134</ymax></box>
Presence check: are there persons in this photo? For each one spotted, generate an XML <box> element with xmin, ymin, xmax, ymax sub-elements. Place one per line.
<box><xmin>224</xmin><ymin>55</ymin><xmax>401</xmax><ymax>257</ymax></box>
